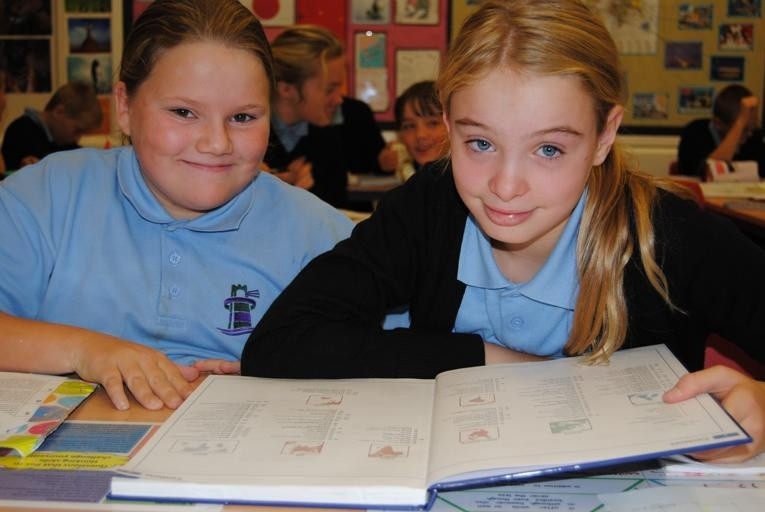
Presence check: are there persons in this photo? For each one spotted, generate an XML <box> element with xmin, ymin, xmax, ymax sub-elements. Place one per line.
<box><xmin>2</xmin><ymin>82</ymin><xmax>103</xmax><ymax>170</ymax></box>
<box><xmin>331</xmin><ymin>93</ymin><xmax>399</xmax><ymax>176</ymax></box>
<box><xmin>263</xmin><ymin>25</ymin><xmax>351</xmax><ymax>209</ymax></box>
<box><xmin>678</xmin><ymin>84</ymin><xmax>765</xmax><ymax>182</ymax></box>
<box><xmin>0</xmin><ymin>0</ymin><xmax>356</xmax><ymax>410</ymax></box>
<box><xmin>240</xmin><ymin>0</ymin><xmax>765</xmax><ymax>464</ymax></box>
<box><xmin>391</xmin><ymin>81</ymin><xmax>450</xmax><ymax>185</ymax></box>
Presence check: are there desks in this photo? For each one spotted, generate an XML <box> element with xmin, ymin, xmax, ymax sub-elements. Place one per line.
<box><xmin>1</xmin><ymin>374</ymin><xmax>765</xmax><ymax>512</ymax></box>
<box><xmin>615</xmin><ymin>125</ymin><xmax>765</xmax><ymax>220</ymax></box>
<box><xmin>338</xmin><ymin>174</ymin><xmax>400</xmax><ymax>224</ymax></box>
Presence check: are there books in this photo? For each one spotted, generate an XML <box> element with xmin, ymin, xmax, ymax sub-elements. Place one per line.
<box><xmin>106</xmin><ymin>344</ymin><xmax>753</xmax><ymax>512</ymax></box>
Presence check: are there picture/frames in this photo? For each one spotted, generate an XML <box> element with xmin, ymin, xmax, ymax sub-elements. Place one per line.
<box><xmin>394</xmin><ymin>46</ymin><xmax>440</xmax><ymax>98</ymax></box>
<box><xmin>353</xmin><ymin>29</ymin><xmax>388</xmax><ymax>115</ymax></box>
<box><xmin>630</xmin><ymin>1</ymin><xmax>763</xmax><ymax>119</ymax></box>
